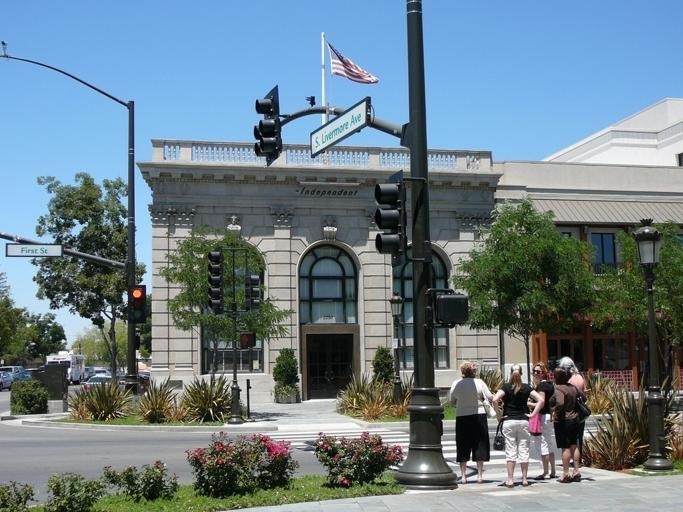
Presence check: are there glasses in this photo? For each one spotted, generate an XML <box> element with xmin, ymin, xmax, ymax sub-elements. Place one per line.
<box><xmin>533</xmin><ymin>369</ymin><xmax>542</xmax><ymax>374</ymax></box>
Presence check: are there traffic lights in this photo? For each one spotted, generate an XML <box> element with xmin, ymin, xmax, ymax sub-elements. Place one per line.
<box><xmin>245</xmin><ymin>268</ymin><xmax>261</xmax><ymax>311</ymax></box>
<box><xmin>240</xmin><ymin>331</ymin><xmax>256</xmax><ymax>351</ymax></box>
<box><xmin>131</xmin><ymin>284</ymin><xmax>146</xmax><ymax>324</ymax></box>
<box><xmin>375</xmin><ymin>170</ymin><xmax>406</xmax><ymax>266</ymax></box>
<box><xmin>253</xmin><ymin>86</ymin><xmax>282</xmax><ymax>166</ymax></box>
<box><xmin>209</xmin><ymin>247</ymin><xmax>225</xmax><ymax>310</ymax></box>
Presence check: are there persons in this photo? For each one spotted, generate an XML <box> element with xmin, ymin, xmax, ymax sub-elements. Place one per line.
<box><xmin>559</xmin><ymin>356</ymin><xmax>588</xmax><ymax>465</ymax></box>
<box><xmin>526</xmin><ymin>361</ymin><xmax>556</xmax><ymax>480</ymax></box>
<box><xmin>492</xmin><ymin>364</ymin><xmax>545</xmax><ymax>488</ymax></box>
<box><xmin>449</xmin><ymin>361</ymin><xmax>495</xmax><ymax>484</ymax></box>
<box><xmin>548</xmin><ymin>367</ymin><xmax>587</xmax><ymax>483</ymax></box>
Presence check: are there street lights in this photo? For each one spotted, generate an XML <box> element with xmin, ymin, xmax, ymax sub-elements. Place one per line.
<box><xmin>632</xmin><ymin>217</ymin><xmax>673</xmax><ymax>471</ymax></box>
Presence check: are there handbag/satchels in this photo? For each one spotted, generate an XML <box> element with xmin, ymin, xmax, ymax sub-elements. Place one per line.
<box><xmin>529</xmin><ymin>412</ymin><xmax>542</xmax><ymax>434</ymax></box>
<box><xmin>494</xmin><ymin>421</ymin><xmax>505</xmax><ymax>450</ymax></box>
<box><xmin>576</xmin><ymin>385</ymin><xmax>591</xmax><ymax>417</ymax></box>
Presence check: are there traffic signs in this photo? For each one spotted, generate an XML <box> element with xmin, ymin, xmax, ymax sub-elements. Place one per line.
<box><xmin>310</xmin><ymin>97</ymin><xmax>370</xmax><ymax>157</ymax></box>
<box><xmin>6</xmin><ymin>244</ymin><xmax>62</xmax><ymax>257</ymax></box>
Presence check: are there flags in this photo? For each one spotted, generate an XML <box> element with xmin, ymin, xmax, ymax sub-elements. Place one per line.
<box><xmin>326</xmin><ymin>41</ymin><xmax>379</xmax><ymax>84</ymax></box>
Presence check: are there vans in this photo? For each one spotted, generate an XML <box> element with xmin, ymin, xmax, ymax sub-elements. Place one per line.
<box><xmin>0</xmin><ymin>365</ymin><xmax>39</xmax><ymax>380</ymax></box>
<box><xmin>0</xmin><ymin>372</ymin><xmax>13</xmax><ymax>389</ymax></box>
<box><xmin>87</xmin><ymin>369</ymin><xmax>113</xmax><ymax>384</ymax></box>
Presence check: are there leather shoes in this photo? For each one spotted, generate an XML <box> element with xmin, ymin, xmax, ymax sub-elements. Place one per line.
<box><xmin>557</xmin><ymin>474</ymin><xmax>581</xmax><ymax>482</ymax></box>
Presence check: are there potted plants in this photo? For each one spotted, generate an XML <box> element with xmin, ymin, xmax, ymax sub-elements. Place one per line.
<box><xmin>272</xmin><ymin>348</ymin><xmax>300</xmax><ymax>404</ymax></box>
<box><xmin>371</xmin><ymin>345</ymin><xmax>394</xmax><ymax>401</ymax></box>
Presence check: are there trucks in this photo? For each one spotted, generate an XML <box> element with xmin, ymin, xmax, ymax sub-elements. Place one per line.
<box><xmin>47</xmin><ymin>355</ymin><xmax>85</xmax><ymax>384</ymax></box>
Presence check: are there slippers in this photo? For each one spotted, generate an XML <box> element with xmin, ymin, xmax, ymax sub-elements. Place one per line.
<box><xmin>535</xmin><ymin>475</ymin><xmax>551</xmax><ymax>480</ymax></box>
<box><xmin>504</xmin><ymin>482</ymin><xmax>513</xmax><ymax>487</ymax></box>
